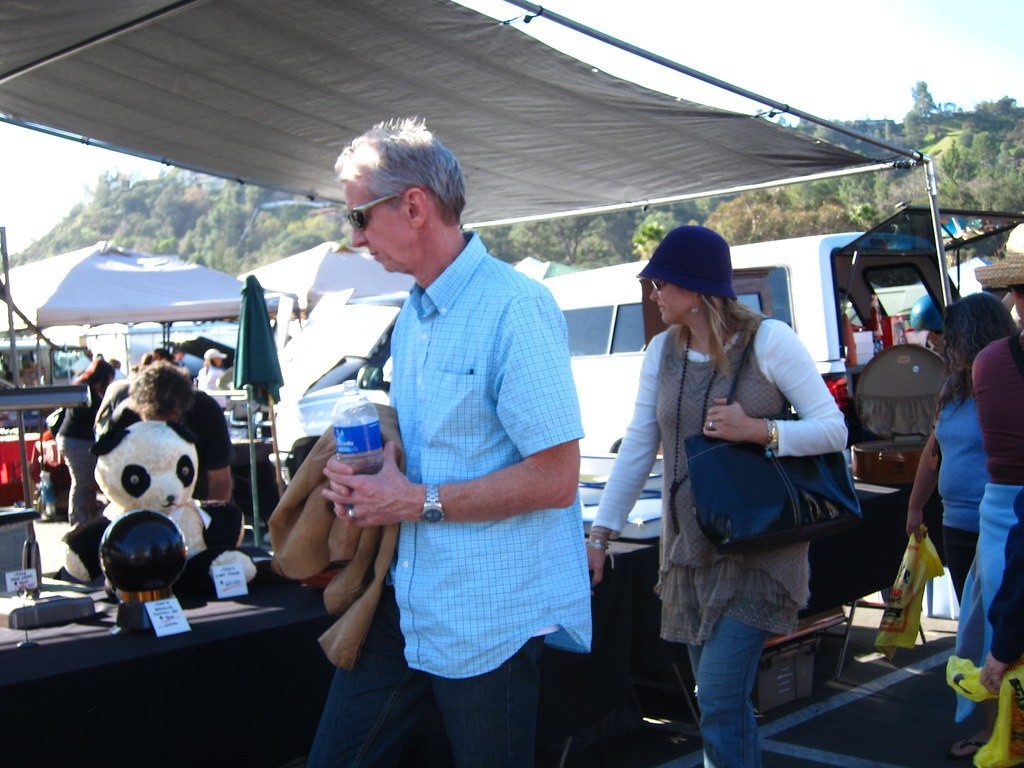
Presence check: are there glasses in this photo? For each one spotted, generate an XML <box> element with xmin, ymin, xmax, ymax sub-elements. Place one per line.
<box><xmin>346</xmin><ymin>194</ymin><xmax>396</xmax><ymax>230</ymax></box>
<box><xmin>651</xmin><ymin>279</ymin><xmax>666</xmax><ymax>290</ymax></box>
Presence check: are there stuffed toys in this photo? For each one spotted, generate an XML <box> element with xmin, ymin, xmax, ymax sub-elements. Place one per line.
<box><xmin>59</xmin><ymin>419</ymin><xmax>253</xmax><ymax>605</ymax></box>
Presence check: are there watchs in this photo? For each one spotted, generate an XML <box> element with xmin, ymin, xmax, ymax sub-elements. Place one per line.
<box><xmin>770</xmin><ymin>422</ymin><xmax>779</xmax><ymax>449</ymax></box>
<box><xmin>423</xmin><ymin>484</ymin><xmax>444</xmax><ymax>525</ymax></box>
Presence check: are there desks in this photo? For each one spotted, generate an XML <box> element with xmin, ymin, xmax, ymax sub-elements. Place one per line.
<box><xmin>0</xmin><ymin>454</ymin><xmax>919</xmax><ymax>768</ymax></box>
<box><xmin>228</xmin><ymin>439</ymin><xmax>277</xmax><ymax>549</ymax></box>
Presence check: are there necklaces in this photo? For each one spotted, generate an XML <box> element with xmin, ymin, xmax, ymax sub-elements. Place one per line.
<box><xmin>670</xmin><ymin>319</ymin><xmax>729</xmax><ymax>533</ymax></box>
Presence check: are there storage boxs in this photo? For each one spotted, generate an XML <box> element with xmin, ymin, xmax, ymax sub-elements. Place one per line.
<box><xmin>849</xmin><ymin>343</ymin><xmax>949</xmax><ymax>485</ymax></box>
<box><xmin>755</xmin><ymin>635</ymin><xmax>823</xmax><ymax>714</ymax></box>
<box><xmin>852</xmin><ymin>330</ymin><xmax>875</xmax><ymax>363</ymax></box>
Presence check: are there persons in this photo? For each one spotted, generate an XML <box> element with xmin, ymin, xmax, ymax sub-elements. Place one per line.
<box><xmin>908</xmin><ymin>221</ymin><xmax>1024</xmax><ymax>768</ymax></box>
<box><xmin>0</xmin><ymin>344</ymin><xmax>234</xmax><ymax>524</ymax></box>
<box><xmin>585</xmin><ymin>226</ymin><xmax>846</xmax><ymax>768</ymax></box>
<box><xmin>306</xmin><ymin>118</ymin><xmax>591</xmax><ymax>768</ymax></box>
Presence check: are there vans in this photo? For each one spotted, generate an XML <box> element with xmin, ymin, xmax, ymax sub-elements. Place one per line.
<box><xmin>269</xmin><ymin>203</ymin><xmax>1023</xmax><ymax>487</ymax></box>
<box><xmin>0</xmin><ymin>347</ymin><xmax>95</xmax><ymax>428</ymax></box>
<box><xmin>846</xmin><ymin>285</ymin><xmax>924</xmax><ymax>328</ymax></box>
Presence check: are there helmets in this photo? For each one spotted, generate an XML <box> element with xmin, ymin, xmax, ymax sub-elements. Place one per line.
<box><xmin>910</xmin><ymin>294</ymin><xmax>942</xmax><ymax>330</ymax></box>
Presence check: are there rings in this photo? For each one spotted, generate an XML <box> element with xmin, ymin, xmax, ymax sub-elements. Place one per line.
<box><xmin>987</xmin><ymin>679</ymin><xmax>992</xmax><ymax>685</ymax></box>
<box><xmin>348</xmin><ymin>510</ymin><xmax>356</xmax><ymax>520</ymax></box>
<box><xmin>709</xmin><ymin>421</ymin><xmax>714</xmax><ymax>430</ymax></box>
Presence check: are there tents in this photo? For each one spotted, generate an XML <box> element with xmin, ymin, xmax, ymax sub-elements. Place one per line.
<box><xmin>0</xmin><ymin>237</ymin><xmax>420</xmax><ymax>390</ymax></box>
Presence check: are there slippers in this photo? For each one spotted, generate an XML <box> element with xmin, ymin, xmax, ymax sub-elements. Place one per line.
<box><xmin>950</xmin><ymin>737</ymin><xmax>986</xmax><ymax>757</ymax></box>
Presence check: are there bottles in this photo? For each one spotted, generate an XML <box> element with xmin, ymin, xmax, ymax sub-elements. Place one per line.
<box><xmin>330</xmin><ymin>380</ymin><xmax>384</xmax><ymax>474</ymax></box>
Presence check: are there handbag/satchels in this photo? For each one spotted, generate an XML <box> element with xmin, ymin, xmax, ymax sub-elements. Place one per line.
<box><xmin>683</xmin><ymin>319</ymin><xmax>864</xmax><ymax>550</ymax></box>
<box><xmin>946</xmin><ymin>654</ymin><xmax>1024</xmax><ymax>768</ymax></box>
<box><xmin>874</xmin><ymin>524</ymin><xmax>945</xmax><ymax>660</ymax></box>
<box><xmin>46</xmin><ymin>407</ymin><xmax>65</xmax><ymax>439</ymax></box>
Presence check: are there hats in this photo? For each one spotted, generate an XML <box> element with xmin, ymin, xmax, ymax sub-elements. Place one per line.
<box><xmin>204</xmin><ymin>349</ymin><xmax>228</xmax><ymax>360</ymax></box>
<box><xmin>637</xmin><ymin>226</ymin><xmax>738</xmax><ymax>299</ymax></box>
<box><xmin>974</xmin><ymin>223</ymin><xmax>1023</xmax><ymax>284</ymax></box>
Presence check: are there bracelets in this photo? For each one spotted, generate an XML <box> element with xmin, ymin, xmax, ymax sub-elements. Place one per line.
<box><xmin>585</xmin><ymin>527</ymin><xmax>615</xmax><ymax>570</ymax></box>
<box><xmin>764</xmin><ymin>418</ymin><xmax>772</xmax><ymax>442</ymax></box>
